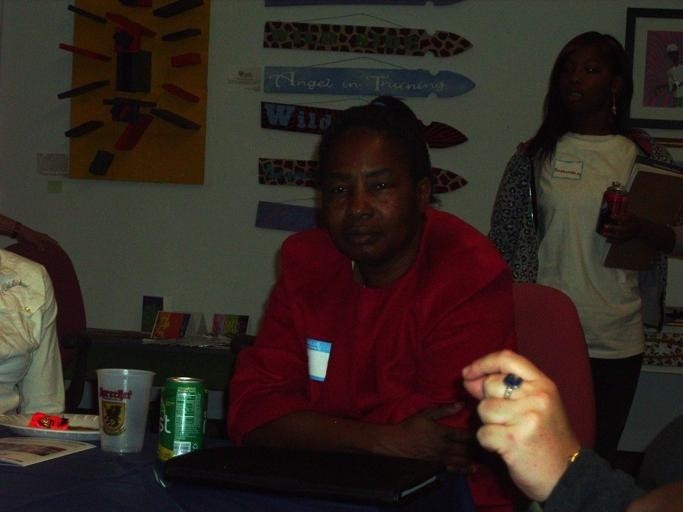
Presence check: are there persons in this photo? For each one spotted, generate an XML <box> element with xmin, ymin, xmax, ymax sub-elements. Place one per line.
<box><xmin>0</xmin><ymin>242</ymin><xmax>67</xmax><ymax>417</ymax></box>
<box><xmin>486</xmin><ymin>30</ymin><xmax>683</xmax><ymax>467</ymax></box>
<box><xmin>665</xmin><ymin>43</ymin><xmax>683</xmax><ymax>106</ymax></box>
<box><xmin>223</xmin><ymin>94</ymin><xmax>518</xmax><ymax>512</ymax></box>
<box><xmin>462</xmin><ymin>349</ymin><xmax>683</xmax><ymax>512</ymax></box>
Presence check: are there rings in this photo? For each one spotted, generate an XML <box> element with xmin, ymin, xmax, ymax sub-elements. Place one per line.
<box><xmin>503</xmin><ymin>373</ymin><xmax>523</xmax><ymax>400</ymax></box>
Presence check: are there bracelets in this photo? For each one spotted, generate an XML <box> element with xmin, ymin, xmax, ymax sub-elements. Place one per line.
<box><xmin>568</xmin><ymin>451</ymin><xmax>579</xmax><ymax>465</ymax></box>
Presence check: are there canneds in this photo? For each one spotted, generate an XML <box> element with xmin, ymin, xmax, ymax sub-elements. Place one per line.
<box><xmin>157</xmin><ymin>376</ymin><xmax>208</xmax><ymax>462</ymax></box>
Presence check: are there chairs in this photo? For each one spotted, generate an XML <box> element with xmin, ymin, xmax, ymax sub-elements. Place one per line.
<box><xmin>470</xmin><ymin>283</ymin><xmax>598</xmax><ymax>512</ymax></box>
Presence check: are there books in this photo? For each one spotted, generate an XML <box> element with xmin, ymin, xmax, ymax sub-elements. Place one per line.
<box><xmin>0</xmin><ymin>435</ymin><xmax>96</xmax><ymax>467</ymax></box>
<box><xmin>141</xmin><ymin>296</ymin><xmax>250</xmax><ymax>350</ymax></box>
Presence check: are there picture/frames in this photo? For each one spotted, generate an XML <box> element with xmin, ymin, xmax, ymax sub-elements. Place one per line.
<box><xmin>626</xmin><ymin>7</ymin><xmax>682</xmax><ymax>127</ymax></box>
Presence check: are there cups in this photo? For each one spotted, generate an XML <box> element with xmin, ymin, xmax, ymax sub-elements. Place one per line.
<box><xmin>96</xmin><ymin>367</ymin><xmax>156</xmax><ymax>456</ymax></box>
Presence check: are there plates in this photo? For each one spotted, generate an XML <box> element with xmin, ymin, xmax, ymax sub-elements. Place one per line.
<box><xmin>0</xmin><ymin>413</ymin><xmax>101</xmax><ymax>442</ymax></box>
